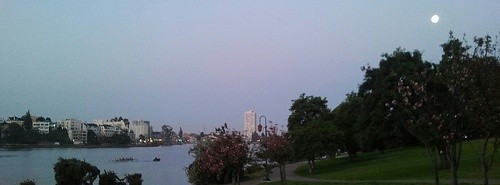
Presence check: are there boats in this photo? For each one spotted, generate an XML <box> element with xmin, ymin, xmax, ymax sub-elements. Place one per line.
<box><xmin>114</xmin><ymin>157</ymin><xmax>134</xmax><ymax>162</ymax></box>
<box><xmin>153</xmin><ymin>157</ymin><xmax>160</xmax><ymax>162</ymax></box>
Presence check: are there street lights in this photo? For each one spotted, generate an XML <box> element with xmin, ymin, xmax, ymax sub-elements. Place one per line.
<box><xmin>258</xmin><ymin>115</ymin><xmax>270</xmax><ymax>182</ymax></box>
